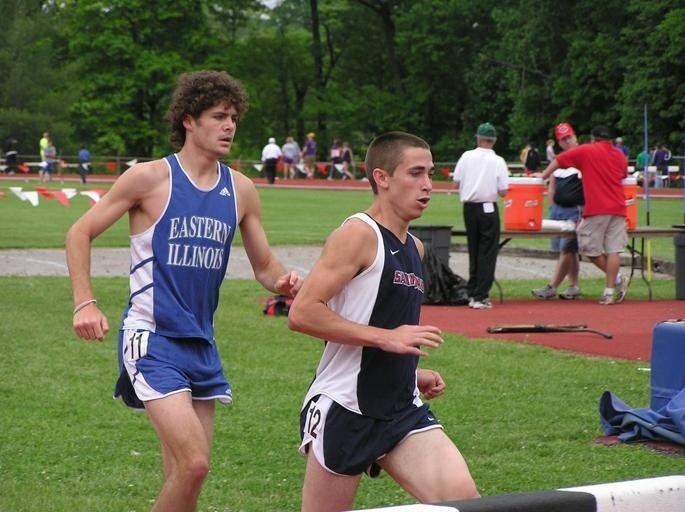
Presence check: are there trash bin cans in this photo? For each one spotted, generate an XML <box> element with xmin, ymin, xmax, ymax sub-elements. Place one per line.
<box><xmin>408</xmin><ymin>225</ymin><xmax>453</xmax><ymax>304</ymax></box>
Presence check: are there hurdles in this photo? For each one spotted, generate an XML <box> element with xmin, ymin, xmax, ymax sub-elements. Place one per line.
<box><xmin>346</xmin><ymin>476</ymin><xmax>685</xmax><ymax>512</ymax></box>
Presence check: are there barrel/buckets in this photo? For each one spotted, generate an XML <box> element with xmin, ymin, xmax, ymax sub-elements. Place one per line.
<box><xmin>621</xmin><ymin>177</ymin><xmax>638</xmax><ymax>230</ymax></box>
<box><xmin>504</xmin><ymin>177</ymin><xmax>548</xmax><ymax>232</ymax></box>
<box><xmin>649</xmin><ymin>319</ymin><xmax>685</xmax><ymax>411</ymax></box>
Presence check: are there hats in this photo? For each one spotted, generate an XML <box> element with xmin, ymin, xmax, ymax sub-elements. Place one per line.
<box><xmin>474</xmin><ymin>122</ymin><xmax>497</xmax><ymax>141</ymax></box>
<box><xmin>553</xmin><ymin>122</ymin><xmax>575</xmax><ymax>140</ymax></box>
<box><xmin>591</xmin><ymin>125</ymin><xmax>610</xmax><ymax>139</ymax></box>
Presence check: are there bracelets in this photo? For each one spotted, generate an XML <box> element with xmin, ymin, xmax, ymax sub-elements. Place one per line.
<box><xmin>73</xmin><ymin>300</ymin><xmax>97</xmax><ymax>315</ymax></box>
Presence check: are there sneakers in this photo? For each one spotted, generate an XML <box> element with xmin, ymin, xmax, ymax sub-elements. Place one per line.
<box><xmin>467</xmin><ymin>297</ymin><xmax>492</xmax><ymax>307</ymax></box>
<box><xmin>616</xmin><ymin>275</ymin><xmax>632</xmax><ymax>304</ymax></box>
<box><xmin>559</xmin><ymin>287</ymin><xmax>582</xmax><ymax>300</ymax></box>
<box><xmin>530</xmin><ymin>284</ymin><xmax>557</xmax><ymax>300</ymax></box>
<box><xmin>473</xmin><ymin>301</ymin><xmax>493</xmax><ymax>310</ymax></box>
<box><xmin>598</xmin><ymin>291</ymin><xmax>616</xmax><ymax>305</ymax></box>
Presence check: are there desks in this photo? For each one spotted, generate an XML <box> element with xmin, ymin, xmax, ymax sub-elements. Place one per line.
<box><xmin>451</xmin><ymin>227</ymin><xmax>684</xmax><ymax>304</ymax></box>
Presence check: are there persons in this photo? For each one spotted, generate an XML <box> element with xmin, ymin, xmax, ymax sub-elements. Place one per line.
<box><xmin>40</xmin><ymin>130</ymin><xmax>56</xmax><ymax>183</ymax></box>
<box><xmin>637</xmin><ymin>144</ymin><xmax>672</xmax><ymax>189</ymax></box>
<box><xmin>5</xmin><ymin>140</ymin><xmax>19</xmax><ymax>173</ymax></box>
<box><xmin>262</xmin><ymin>133</ymin><xmax>355</xmax><ymax>184</ymax></box>
<box><xmin>453</xmin><ymin>123</ymin><xmax>509</xmax><ymax>309</ymax></box>
<box><xmin>519</xmin><ymin>122</ymin><xmax>631</xmax><ymax>306</ymax></box>
<box><xmin>66</xmin><ymin>68</ymin><xmax>301</xmax><ymax>512</ymax></box>
<box><xmin>79</xmin><ymin>144</ymin><xmax>90</xmax><ymax>183</ymax></box>
<box><xmin>286</xmin><ymin>132</ymin><xmax>480</xmax><ymax>512</ymax></box>
